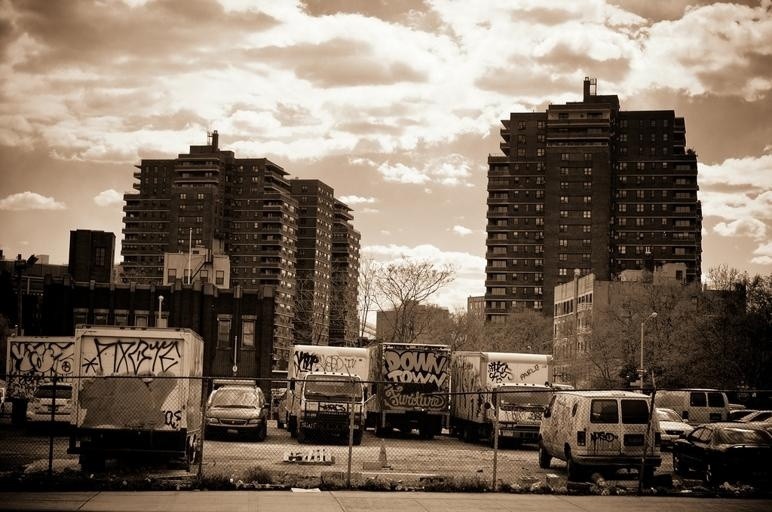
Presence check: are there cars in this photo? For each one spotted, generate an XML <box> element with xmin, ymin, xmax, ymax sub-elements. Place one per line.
<box><xmin>651</xmin><ymin>388</ymin><xmax>772</xmax><ymax>485</ymax></box>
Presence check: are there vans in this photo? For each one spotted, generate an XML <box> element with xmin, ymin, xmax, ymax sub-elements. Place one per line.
<box><xmin>538</xmin><ymin>390</ymin><xmax>662</xmax><ymax>482</ymax></box>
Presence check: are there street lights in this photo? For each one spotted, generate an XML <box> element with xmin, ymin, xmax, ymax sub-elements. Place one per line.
<box><xmin>640</xmin><ymin>312</ymin><xmax>657</xmax><ymax>393</ymax></box>
<box><xmin>158</xmin><ymin>296</ymin><xmax>164</xmax><ymax>328</ymax></box>
